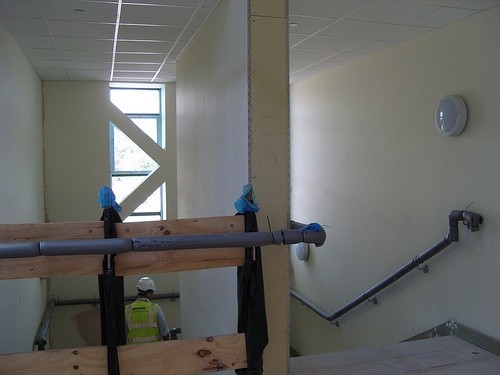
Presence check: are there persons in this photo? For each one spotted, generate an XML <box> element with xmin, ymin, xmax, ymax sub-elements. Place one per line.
<box><xmin>125</xmin><ymin>278</ymin><xmax>169</xmax><ymax>344</ymax></box>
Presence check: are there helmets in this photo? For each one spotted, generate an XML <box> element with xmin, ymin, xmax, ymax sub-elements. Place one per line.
<box><xmin>137</xmin><ymin>277</ymin><xmax>157</xmax><ymax>291</ymax></box>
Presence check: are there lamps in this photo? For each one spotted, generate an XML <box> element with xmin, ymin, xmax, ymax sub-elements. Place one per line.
<box><xmin>434</xmin><ymin>94</ymin><xmax>468</xmax><ymax>137</ymax></box>
<box><xmin>295</xmin><ymin>242</ymin><xmax>309</xmax><ymax>261</ymax></box>
